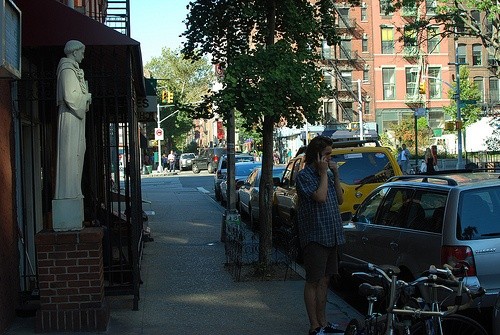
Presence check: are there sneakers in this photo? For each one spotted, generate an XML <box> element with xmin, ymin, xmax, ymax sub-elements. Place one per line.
<box><xmin>308</xmin><ymin>326</ymin><xmax>326</xmax><ymax>335</ymax></box>
<box><xmin>320</xmin><ymin>321</ymin><xmax>345</xmax><ymax>335</ymax></box>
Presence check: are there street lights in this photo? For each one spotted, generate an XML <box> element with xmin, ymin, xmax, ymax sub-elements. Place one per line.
<box><xmin>405</xmin><ymin>102</ymin><xmax>426</xmax><ymax>173</ymax></box>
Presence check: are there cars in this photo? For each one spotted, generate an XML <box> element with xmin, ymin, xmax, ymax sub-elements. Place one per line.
<box><xmin>175</xmin><ymin>152</ymin><xmax>195</xmax><ymax>171</ymax></box>
<box><xmin>237</xmin><ymin>163</ymin><xmax>290</xmax><ymax>227</ymax></box>
<box><xmin>217</xmin><ymin>161</ymin><xmax>263</xmax><ymax>208</ymax></box>
<box><xmin>190</xmin><ymin>147</ymin><xmax>227</xmax><ymax>175</ymax></box>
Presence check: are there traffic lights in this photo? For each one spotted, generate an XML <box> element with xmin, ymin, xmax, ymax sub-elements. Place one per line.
<box><xmin>162</xmin><ymin>91</ymin><xmax>168</xmax><ymax>104</ymax></box>
<box><xmin>418</xmin><ymin>81</ymin><xmax>425</xmax><ymax>95</ymax></box>
<box><xmin>168</xmin><ymin>92</ymin><xmax>174</xmax><ymax>104</ymax></box>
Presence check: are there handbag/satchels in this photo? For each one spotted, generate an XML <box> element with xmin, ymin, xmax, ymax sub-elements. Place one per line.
<box><xmin>421</xmin><ymin>163</ymin><xmax>427</xmax><ymax>173</ymax></box>
<box><xmin>434</xmin><ymin>164</ymin><xmax>440</xmax><ymax>171</ymax></box>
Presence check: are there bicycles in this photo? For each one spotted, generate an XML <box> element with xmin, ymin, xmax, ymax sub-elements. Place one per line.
<box><xmin>337</xmin><ymin>259</ymin><xmax>491</xmax><ymax>335</ymax></box>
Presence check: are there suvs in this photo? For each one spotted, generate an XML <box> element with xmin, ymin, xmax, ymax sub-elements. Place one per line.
<box><xmin>214</xmin><ymin>154</ymin><xmax>255</xmax><ymax>200</ymax></box>
<box><xmin>273</xmin><ymin>129</ymin><xmax>409</xmax><ymax>264</ymax></box>
<box><xmin>341</xmin><ymin>170</ymin><xmax>500</xmax><ymax>304</ymax></box>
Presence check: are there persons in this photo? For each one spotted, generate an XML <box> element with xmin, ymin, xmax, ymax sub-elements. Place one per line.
<box><xmin>168</xmin><ymin>150</ymin><xmax>175</xmax><ymax>172</ymax></box>
<box><xmin>54</xmin><ymin>40</ymin><xmax>92</xmax><ymax>199</ymax></box>
<box><xmin>431</xmin><ymin>146</ymin><xmax>439</xmax><ymax>171</ymax></box>
<box><xmin>295</xmin><ymin>136</ymin><xmax>343</xmax><ymax>335</ymax></box>
<box><xmin>143</xmin><ymin>153</ymin><xmax>179</xmax><ymax>170</ymax></box>
<box><xmin>249</xmin><ymin>149</ymin><xmax>298</xmax><ymax>165</ymax></box>
<box><xmin>421</xmin><ymin>148</ymin><xmax>434</xmax><ymax>173</ymax></box>
<box><xmin>399</xmin><ymin>144</ymin><xmax>410</xmax><ymax>175</ymax></box>
